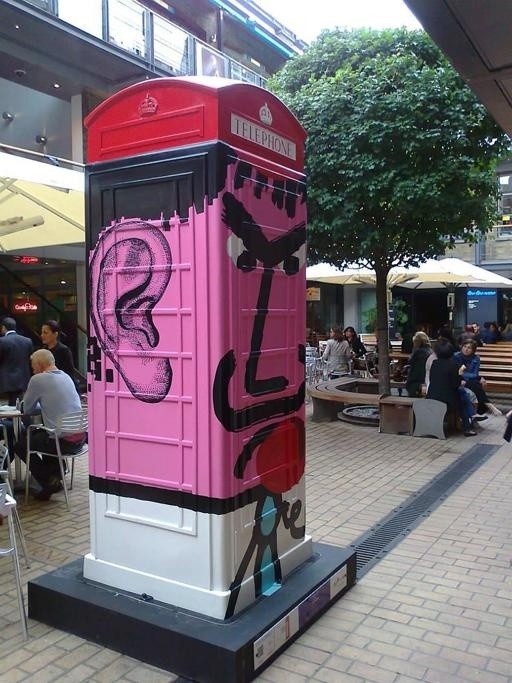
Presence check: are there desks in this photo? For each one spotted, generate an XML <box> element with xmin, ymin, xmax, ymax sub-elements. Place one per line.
<box><xmin>1</xmin><ymin>408</ymin><xmax>40</xmax><ymax>490</ymax></box>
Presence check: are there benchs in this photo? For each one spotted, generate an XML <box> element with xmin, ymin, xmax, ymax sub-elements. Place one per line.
<box><xmin>306</xmin><ymin>375</ymin><xmax>407</xmax><ymax>423</ymax></box>
<box><xmin>465</xmin><ymin>340</ymin><xmax>511</xmax><ymax>394</ymax></box>
<box><xmin>377</xmin><ymin>392</ymin><xmax>465</xmax><ymax>440</ymax></box>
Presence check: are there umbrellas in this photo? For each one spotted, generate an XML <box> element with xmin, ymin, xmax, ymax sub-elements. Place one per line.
<box><xmin>305</xmin><ymin>248</ymin><xmax>512</xmax><ymax>348</ymax></box>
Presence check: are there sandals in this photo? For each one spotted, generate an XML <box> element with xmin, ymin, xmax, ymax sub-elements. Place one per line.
<box><xmin>469</xmin><ymin>414</ymin><xmax>489</xmax><ymax>421</ymax></box>
<box><xmin>463</xmin><ymin>431</ymin><xmax>478</xmax><ymax>436</ymax></box>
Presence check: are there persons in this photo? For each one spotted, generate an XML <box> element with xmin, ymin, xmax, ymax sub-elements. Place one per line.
<box><xmin>401</xmin><ymin>316</ymin><xmax>512</xmax><ymax>444</ymax></box>
<box><xmin>40</xmin><ymin>320</ymin><xmax>83</xmax><ymax>396</ymax></box>
<box><xmin>0</xmin><ymin>416</ymin><xmax>18</xmax><ymax>470</ymax></box>
<box><xmin>0</xmin><ymin>318</ymin><xmax>35</xmax><ymax>406</ymax></box>
<box><xmin>15</xmin><ymin>350</ymin><xmax>86</xmax><ymax>501</ymax></box>
<box><xmin>322</xmin><ymin>327</ymin><xmax>354</xmax><ymax>379</ymax></box>
<box><xmin>343</xmin><ymin>327</ymin><xmax>367</xmax><ymax>357</ymax></box>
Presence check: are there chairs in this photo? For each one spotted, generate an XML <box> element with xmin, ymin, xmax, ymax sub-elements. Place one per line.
<box><xmin>0</xmin><ymin>443</ymin><xmax>30</xmax><ymax>641</ymax></box>
<box><xmin>22</xmin><ymin>408</ymin><xmax>89</xmax><ymax>512</ymax></box>
<box><xmin>305</xmin><ymin>339</ymin><xmax>329</xmax><ymax>402</ymax></box>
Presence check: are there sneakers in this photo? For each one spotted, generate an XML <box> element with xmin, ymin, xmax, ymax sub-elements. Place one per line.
<box><xmin>33</xmin><ymin>473</ymin><xmax>64</xmax><ymax>501</ymax></box>
